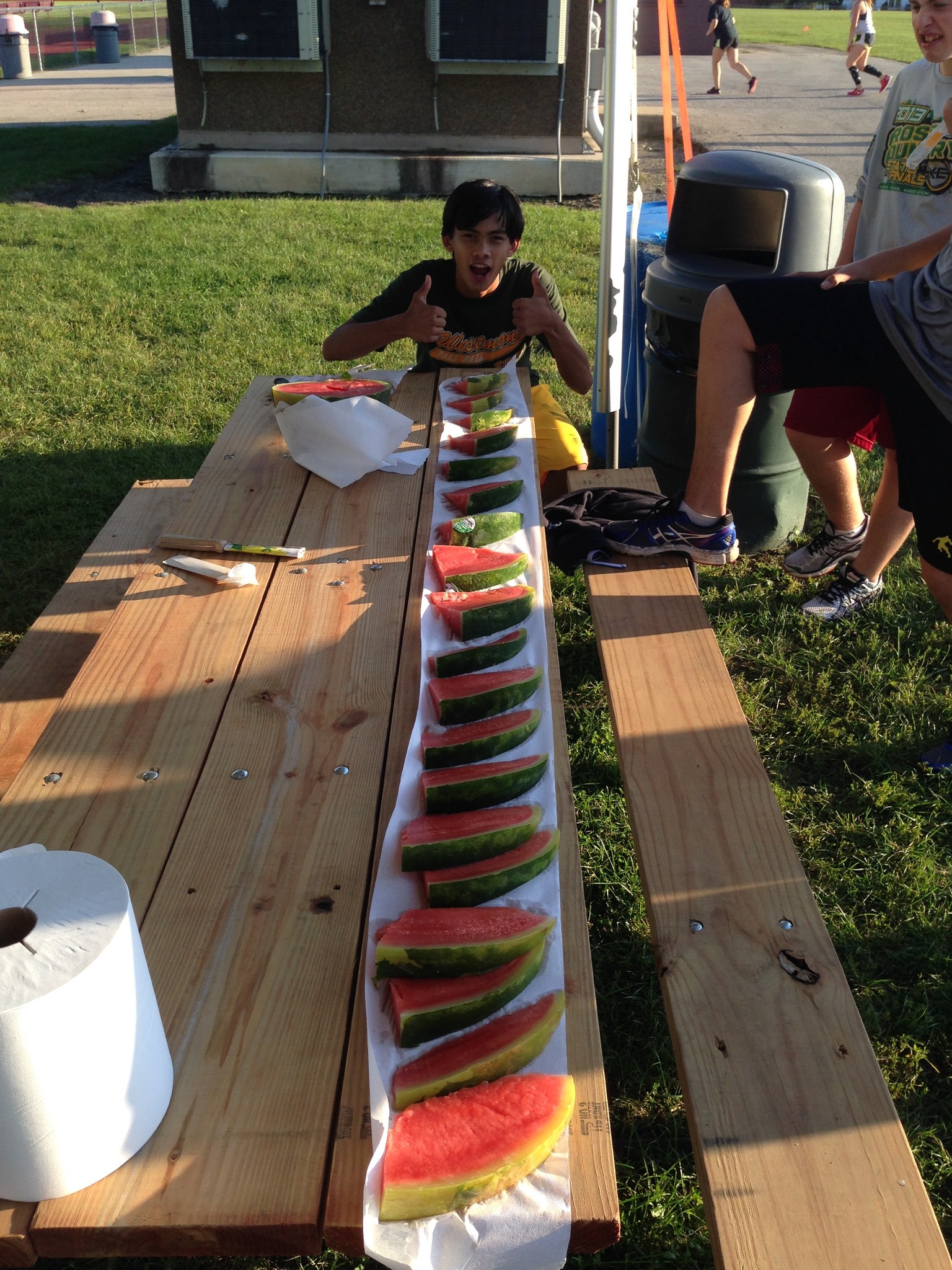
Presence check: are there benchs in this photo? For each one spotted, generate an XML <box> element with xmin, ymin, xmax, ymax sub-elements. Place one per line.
<box><xmin>568</xmin><ymin>462</ymin><xmax>952</xmax><ymax>1270</ymax></box>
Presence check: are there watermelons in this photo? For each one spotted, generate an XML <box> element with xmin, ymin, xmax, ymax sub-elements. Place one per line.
<box><xmin>377</xmin><ymin>1073</ymin><xmax>576</xmax><ymax>1218</ymax></box>
<box><xmin>399</xmin><ymin>754</ymin><xmax>559</xmax><ymax>909</ymax></box>
<box><xmin>373</xmin><ymin>904</ymin><xmax>558</xmax><ymax>1047</ymax></box>
<box><xmin>272</xmin><ymin>379</ymin><xmax>393</xmax><ymax>408</ymax></box>
<box><xmin>392</xmin><ymin>988</ymin><xmax>567</xmax><ymax>1110</ymax></box>
<box><xmin>445</xmin><ymin>372</ymin><xmax>519</xmax><ymax>458</ymax></box>
<box><xmin>421</xmin><ymin>543</ymin><xmax>542</xmax><ymax>768</ymax></box>
<box><xmin>436</xmin><ymin>455</ymin><xmax>524</xmax><ymax>548</ymax></box>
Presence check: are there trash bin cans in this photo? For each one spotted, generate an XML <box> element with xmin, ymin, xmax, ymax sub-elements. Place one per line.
<box><xmin>0</xmin><ymin>14</ymin><xmax>33</xmax><ymax>79</ymax></box>
<box><xmin>633</xmin><ymin>150</ymin><xmax>847</xmax><ymax>552</ymax></box>
<box><xmin>90</xmin><ymin>10</ymin><xmax>121</xmax><ymax>63</ymax></box>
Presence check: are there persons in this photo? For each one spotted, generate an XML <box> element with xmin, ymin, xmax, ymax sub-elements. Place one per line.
<box><xmin>845</xmin><ymin>0</ymin><xmax>893</xmax><ymax>96</ymax></box>
<box><xmin>780</xmin><ymin>0</ymin><xmax>952</xmax><ymax>620</ymax></box>
<box><xmin>322</xmin><ymin>178</ymin><xmax>593</xmax><ymax>512</ymax></box>
<box><xmin>602</xmin><ymin>223</ymin><xmax>952</xmax><ymax>773</ymax></box>
<box><xmin>706</xmin><ymin>0</ymin><xmax>758</xmax><ymax>94</ymax></box>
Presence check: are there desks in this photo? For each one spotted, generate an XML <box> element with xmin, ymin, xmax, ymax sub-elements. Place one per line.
<box><xmin>0</xmin><ymin>362</ymin><xmax>626</xmax><ymax>1270</ymax></box>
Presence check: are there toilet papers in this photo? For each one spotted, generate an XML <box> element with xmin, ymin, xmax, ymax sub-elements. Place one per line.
<box><xmin>1</xmin><ymin>842</ymin><xmax>177</xmax><ymax>1201</ymax></box>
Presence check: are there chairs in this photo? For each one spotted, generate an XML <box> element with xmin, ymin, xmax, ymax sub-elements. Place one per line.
<box><xmin>0</xmin><ymin>475</ymin><xmax>196</xmax><ymax>799</ymax></box>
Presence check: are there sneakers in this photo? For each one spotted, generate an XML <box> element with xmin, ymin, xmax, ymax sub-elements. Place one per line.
<box><xmin>707</xmin><ymin>87</ymin><xmax>721</xmax><ymax>94</ymax></box>
<box><xmin>601</xmin><ymin>489</ymin><xmax>740</xmax><ymax>566</ymax></box>
<box><xmin>798</xmin><ymin>564</ymin><xmax>883</xmax><ymax>622</ymax></box>
<box><xmin>921</xmin><ymin>739</ymin><xmax>952</xmax><ymax>769</ymax></box>
<box><xmin>879</xmin><ymin>74</ymin><xmax>892</xmax><ymax>93</ymax></box>
<box><xmin>747</xmin><ymin>77</ymin><xmax>758</xmax><ymax>94</ymax></box>
<box><xmin>847</xmin><ymin>88</ymin><xmax>866</xmax><ymax>96</ymax></box>
<box><xmin>782</xmin><ymin>513</ymin><xmax>870</xmax><ymax>579</ymax></box>
